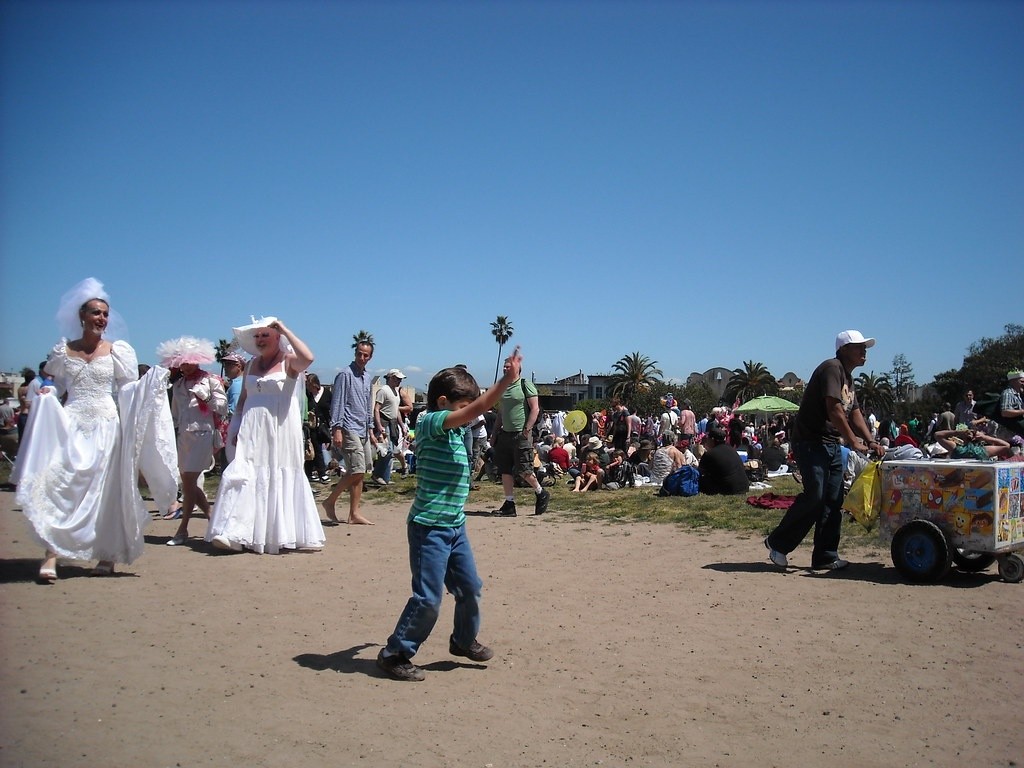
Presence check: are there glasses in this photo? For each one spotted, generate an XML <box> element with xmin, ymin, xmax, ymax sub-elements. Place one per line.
<box><xmin>225</xmin><ymin>363</ymin><xmax>239</xmax><ymax>367</ymax></box>
<box><xmin>612</xmin><ymin>404</ymin><xmax>617</xmax><ymax>408</ymax></box>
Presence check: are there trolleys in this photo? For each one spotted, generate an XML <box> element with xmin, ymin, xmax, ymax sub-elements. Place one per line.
<box><xmin>852</xmin><ymin>448</ymin><xmax>1024</xmax><ymax>585</ymax></box>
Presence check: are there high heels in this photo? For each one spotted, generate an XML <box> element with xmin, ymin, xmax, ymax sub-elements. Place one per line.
<box><xmin>38</xmin><ymin>562</ymin><xmax>57</xmax><ymax>580</ymax></box>
<box><xmin>91</xmin><ymin>561</ymin><xmax>115</xmax><ymax>575</ymax></box>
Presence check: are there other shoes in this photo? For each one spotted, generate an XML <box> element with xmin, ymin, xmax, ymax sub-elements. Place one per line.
<box><xmin>305</xmin><ymin>464</ymin><xmax>410</xmax><ymax>485</ymax></box>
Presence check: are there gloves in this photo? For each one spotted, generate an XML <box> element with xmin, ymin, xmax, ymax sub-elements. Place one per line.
<box><xmin>189</xmin><ymin>382</ymin><xmax>209</xmax><ymax>401</ymax></box>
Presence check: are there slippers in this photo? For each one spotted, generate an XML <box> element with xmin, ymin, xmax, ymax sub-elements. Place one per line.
<box><xmin>163</xmin><ymin>500</ymin><xmax>180</xmax><ymax>520</ymax></box>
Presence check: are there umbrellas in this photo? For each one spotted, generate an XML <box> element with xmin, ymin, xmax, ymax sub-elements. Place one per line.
<box><xmin>735</xmin><ymin>393</ymin><xmax>799</xmax><ymax>438</ymax></box>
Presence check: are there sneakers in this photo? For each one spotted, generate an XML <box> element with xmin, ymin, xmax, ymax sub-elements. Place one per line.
<box><xmin>765</xmin><ymin>538</ymin><xmax>788</xmax><ymax>570</ymax></box>
<box><xmin>167</xmin><ymin>532</ymin><xmax>188</xmax><ymax>546</ymax></box>
<box><xmin>535</xmin><ymin>487</ymin><xmax>550</xmax><ymax>515</ymax></box>
<box><xmin>449</xmin><ymin>633</ymin><xmax>495</xmax><ymax>661</ymax></box>
<box><xmin>212</xmin><ymin>535</ymin><xmax>242</xmax><ymax>551</ymax></box>
<box><xmin>377</xmin><ymin>648</ymin><xmax>425</xmax><ymax>681</ymax></box>
<box><xmin>814</xmin><ymin>557</ymin><xmax>850</xmax><ymax>569</ymax></box>
<box><xmin>491</xmin><ymin>500</ymin><xmax>517</xmax><ymax>517</ymax></box>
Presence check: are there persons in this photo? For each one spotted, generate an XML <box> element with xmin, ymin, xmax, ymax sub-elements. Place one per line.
<box><xmin>204</xmin><ymin>316</ymin><xmax>326</xmax><ymax>555</ymax></box>
<box><xmin>157</xmin><ymin>336</ymin><xmax>227</xmax><ymax>546</ymax></box>
<box><xmin>322</xmin><ymin>330</ymin><xmax>378</xmax><ymax>526</ymax></box>
<box><xmin>8</xmin><ymin>276</ymin><xmax>179</xmax><ymax>578</ymax></box>
<box><xmin>765</xmin><ymin>329</ymin><xmax>886</xmax><ymax>571</ymax></box>
<box><xmin>376</xmin><ymin>344</ymin><xmax>523</xmax><ymax>682</ymax></box>
<box><xmin>515</xmin><ymin>399</ymin><xmax>797</xmax><ymax>494</ymax></box>
<box><xmin>490</xmin><ymin>357</ymin><xmax>549</xmax><ymax>516</ymax></box>
<box><xmin>840</xmin><ymin>370</ymin><xmax>1024</xmax><ymax>493</ymax></box>
<box><xmin>0</xmin><ymin>352</ymin><xmax>499</xmax><ymax>488</ymax></box>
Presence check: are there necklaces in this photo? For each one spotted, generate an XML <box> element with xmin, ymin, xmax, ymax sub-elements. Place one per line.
<box><xmin>80</xmin><ymin>339</ymin><xmax>104</xmax><ymax>361</ymax></box>
<box><xmin>261</xmin><ymin>350</ymin><xmax>280</xmax><ymax>373</ymax></box>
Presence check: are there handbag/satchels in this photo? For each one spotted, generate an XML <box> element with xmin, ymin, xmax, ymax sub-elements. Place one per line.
<box><xmin>661</xmin><ymin>464</ymin><xmax>699</xmax><ymax>496</ymax></box>
<box><xmin>390</xmin><ymin>419</ymin><xmax>399</xmax><ymax>446</ymax></box>
<box><xmin>214</xmin><ymin>428</ymin><xmax>223</xmax><ymax>448</ymax></box>
<box><xmin>671</xmin><ymin>425</ymin><xmax>676</xmax><ymax>432</ymax></box>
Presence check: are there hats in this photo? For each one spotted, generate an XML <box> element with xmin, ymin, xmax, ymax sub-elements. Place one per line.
<box><xmin>387</xmin><ymin>370</ymin><xmax>406</xmax><ymax>379</ymax></box>
<box><xmin>639</xmin><ymin>439</ymin><xmax>652</xmax><ymax>449</ymax></box>
<box><xmin>156</xmin><ymin>315</ymin><xmax>295</xmax><ymax>368</ymax></box>
<box><xmin>836</xmin><ymin>329</ymin><xmax>875</xmax><ymax>353</ymax></box>
<box><xmin>1007</xmin><ymin>369</ymin><xmax>1024</xmax><ymax>380</ymax></box>
<box><xmin>587</xmin><ymin>436</ymin><xmax>603</xmax><ymax>450</ymax></box>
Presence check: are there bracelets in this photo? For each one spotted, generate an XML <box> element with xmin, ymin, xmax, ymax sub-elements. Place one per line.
<box><xmin>868</xmin><ymin>441</ymin><xmax>878</xmax><ymax>445</ymax></box>
